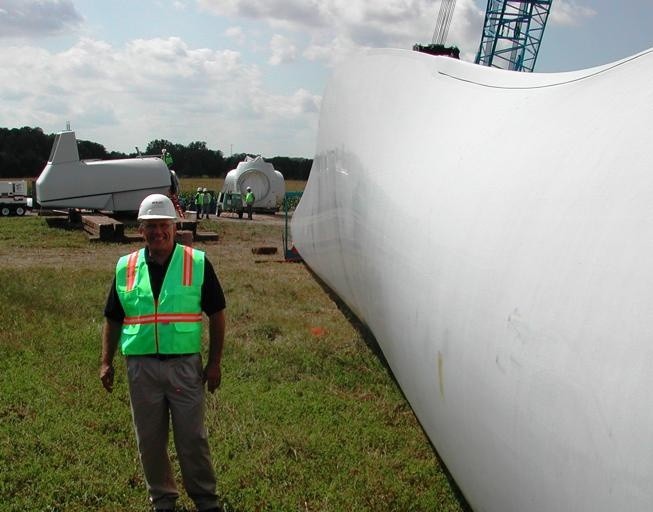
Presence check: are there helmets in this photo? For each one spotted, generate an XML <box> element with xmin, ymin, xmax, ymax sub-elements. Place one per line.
<box><xmin>136</xmin><ymin>193</ymin><xmax>179</xmax><ymax>222</ymax></box>
<box><xmin>196</xmin><ymin>187</ymin><xmax>208</xmax><ymax>193</ymax></box>
<box><xmin>245</xmin><ymin>186</ymin><xmax>252</xmax><ymax>190</ymax></box>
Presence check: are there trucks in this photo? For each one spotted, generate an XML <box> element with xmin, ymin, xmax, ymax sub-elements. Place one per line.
<box><xmin>0</xmin><ymin>180</ymin><xmax>27</xmax><ymax>216</ymax></box>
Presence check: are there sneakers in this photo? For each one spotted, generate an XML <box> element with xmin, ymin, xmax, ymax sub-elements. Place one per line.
<box><xmin>196</xmin><ymin>215</ymin><xmax>211</xmax><ymax>220</ymax></box>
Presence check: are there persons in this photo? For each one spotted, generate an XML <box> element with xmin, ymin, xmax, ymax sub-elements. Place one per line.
<box><xmin>244</xmin><ymin>186</ymin><xmax>255</xmax><ymax>220</ymax></box>
<box><xmin>200</xmin><ymin>188</ymin><xmax>211</xmax><ymax>219</ymax></box>
<box><xmin>159</xmin><ymin>149</ymin><xmax>173</xmax><ymax>169</ymax></box>
<box><xmin>97</xmin><ymin>193</ymin><xmax>228</xmax><ymax>511</ymax></box>
<box><xmin>193</xmin><ymin>187</ymin><xmax>202</xmax><ymax>220</ymax></box>
